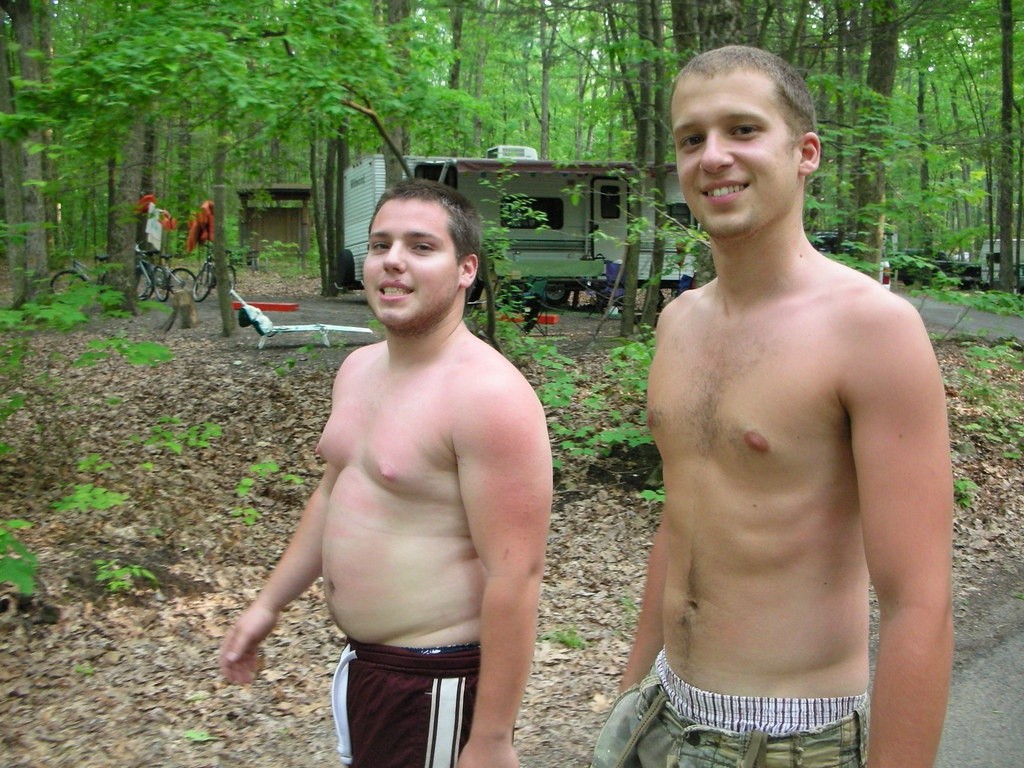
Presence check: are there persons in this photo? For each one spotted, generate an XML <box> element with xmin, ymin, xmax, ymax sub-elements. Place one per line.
<box><xmin>596</xmin><ymin>45</ymin><xmax>955</xmax><ymax>767</ymax></box>
<box><xmin>218</xmin><ymin>177</ymin><xmax>552</xmax><ymax>768</ymax></box>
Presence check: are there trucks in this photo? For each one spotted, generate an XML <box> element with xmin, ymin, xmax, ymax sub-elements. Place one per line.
<box><xmin>336</xmin><ymin>145</ymin><xmax>718</xmax><ymax>323</ymax></box>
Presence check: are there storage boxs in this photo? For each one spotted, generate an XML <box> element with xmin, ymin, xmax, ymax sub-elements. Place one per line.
<box><xmin>525</xmin><ymin>313</ymin><xmax>560</xmax><ymax>323</ymax></box>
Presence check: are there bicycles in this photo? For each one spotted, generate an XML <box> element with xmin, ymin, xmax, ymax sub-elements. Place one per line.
<box><xmin>132</xmin><ymin>248</ymin><xmax>199</xmax><ymax>303</ymax></box>
<box><xmin>191</xmin><ymin>242</ymin><xmax>237</xmax><ymax>302</ymax></box>
<box><xmin>131</xmin><ymin>240</ymin><xmax>171</xmax><ymax>303</ymax></box>
<box><xmin>50</xmin><ymin>250</ymin><xmax>126</xmax><ymax>305</ymax></box>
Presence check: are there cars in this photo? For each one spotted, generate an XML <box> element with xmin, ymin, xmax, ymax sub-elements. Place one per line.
<box><xmin>812</xmin><ymin>228</ymin><xmax>1024</xmax><ymax>297</ymax></box>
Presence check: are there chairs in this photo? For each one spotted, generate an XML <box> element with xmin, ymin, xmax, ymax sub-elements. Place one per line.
<box><xmin>496</xmin><ymin>275</ymin><xmax>550</xmax><ymax>339</ymax></box>
<box><xmin>229</xmin><ymin>288</ymin><xmax>373</xmax><ymax>350</ymax></box>
<box><xmin>584</xmin><ymin>259</ymin><xmax>624</xmax><ymax>316</ymax></box>
